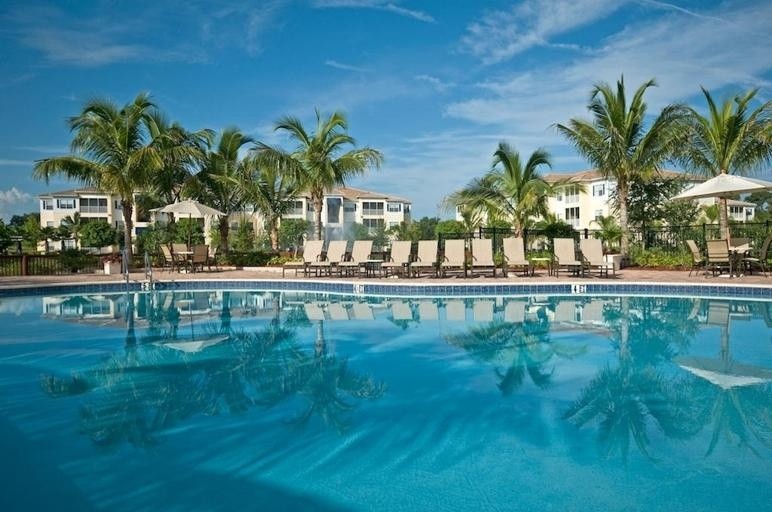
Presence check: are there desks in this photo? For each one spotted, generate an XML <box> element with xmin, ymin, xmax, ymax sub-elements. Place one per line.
<box><xmin>531</xmin><ymin>257</ymin><xmax>551</xmax><ymax>278</ymax></box>
<box><xmin>358</xmin><ymin>258</ymin><xmax>386</xmax><ymax>280</ymax></box>
<box><xmin>528</xmin><ymin>296</ymin><xmax>552</xmax><ymax>307</ymax></box>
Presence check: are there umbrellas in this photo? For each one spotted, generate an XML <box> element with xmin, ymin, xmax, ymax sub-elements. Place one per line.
<box><xmin>147</xmin><ymin>197</ymin><xmax>228</xmax><ymax>253</ymax></box>
<box><xmin>668</xmin><ymin>170</ymin><xmax>772</xmax><ymax>250</ymax></box>
<box><xmin>677</xmin><ymin>317</ymin><xmax>772</xmax><ymax>391</ymax></box>
<box><xmin>152</xmin><ymin>302</ymin><xmax>229</xmax><ymax>355</ymax></box>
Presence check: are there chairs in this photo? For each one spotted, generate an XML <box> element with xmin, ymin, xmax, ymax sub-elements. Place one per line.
<box><xmin>301</xmin><ymin>301</ymin><xmax>326</xmax><ymax>322</ymax></box>
<box><xmin>443</xmin><ymin>299</ymin><xmax>467</xmax><ymax>322</ymax></box>
<box><xmin>552</xmin><ymin>238</ymin><xmax>585</xmax><ymax>279</ymax></box>
<box><xmin>686</xmin><ymin>299</ymin><xmax>753</xmax><ymax>328</ymax></box>
<box><xmin>159</xmin><ymin>242</ymin><xmax>219</xmax><ymax>274</ymax></box>
<box><xmin>336</xmin><ymin>240</ymin><xmax>374</xmax><ymax>279</ymax></box>
<box><xmin>353</xmin><ymin>303</ymin><xmax>376</xmax><ymax>322</ymax></box>
<box><xmin>440</xmin><ymin>240</ymin><xmax>468</xmax><ymax>278</ymax></box>
<box><xmin>579</xmin><ymin>238</ymin><xmax>610</xmax><ymax>279</ymax></box>
<box><xmin>503</xmin><ymin>300</ymin><xmax>527</xmax><ymax>324</ymax></box>
<box><xmin>552</xmin><ymin>297</ymin><xmax>605</xmax><ymax>331</ymax></box>
<box><xmin>159</xmin><ymin>293</ymin><xmax>221</xmax><ymax>318</ymax></box>
<box><xmin>410</xmin><ymin>240</ymin><xmax>439</xmax><ymax>279</ymax></box>
<box><xmin>284</xmin><ymin>240</ymin><xmax>325</xmax><ymax>278</ymax></box>
<box><xmin>381</xmin><ymin>240</ymin><xmax>413</xmax><ymax>278</ymax></box>
<box><xmin>471</xmin><ymin>299</ymin><xmax>495</xmax><ymax>323</ymax></box>
<box><xmin>470</xmin><ymin>239</ymin><xmax>497</xmax><ymax>279</ymax></box>
<box><xmin>309</xmin><ymin>240</ymin><xmax>349</xmax><ymax>278</ymax></box>
<box><xmin>385</xmin><ymin>300</ymin><xmax>413</xmax><ymax>321</ymax></box>
<box><xmin>411</xmin><ymin>300</ymin><xmax>440</xmax><ymax>321</ymax></box>
<box><xmin>501</xmin><ymin>237</ymin><xmax>530</xmax><ymax>279</ymax></box>
<box><xmin>686</xmin><ymin>234</ymin><xmax>772</xmax><ymax>279</ymax></box>
<box><xmin>326</xmin><ymin>302</ymin><xmax>351</xmax><ymax>322</ymax></box>
